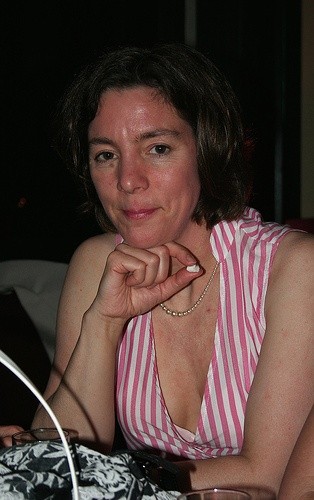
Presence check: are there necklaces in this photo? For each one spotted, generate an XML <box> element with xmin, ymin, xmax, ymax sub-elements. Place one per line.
<box><xmin>158</xmin><ymin>257</ymin><xmax>221</xmax><ymax>316</ymax></box>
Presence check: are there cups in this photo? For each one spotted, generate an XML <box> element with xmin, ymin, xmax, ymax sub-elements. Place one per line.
<box><xmin>177</xmin><ymin>488</ymin><xmax>252</xmax><ymax>500</ymax></box>
<box><xmin>12</xmin><ymin>428</ymin><xmax>70</xmax><ymax>445</ymax></box>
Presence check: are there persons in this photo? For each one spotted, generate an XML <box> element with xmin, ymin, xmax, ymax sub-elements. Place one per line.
<box><xmin>278</xmin><ymin>406</ymin><xmax>314</xmax><ymax>499</ymax></box>
<box><xmin>0</xmin><ymin>425</ymin><xmax>32</xmax><ymax>450</ymax></box>
<box><xmin>28</xmin><ymin>43</ymin><xmax>314</xmax><ymax>492</ymax></box>
